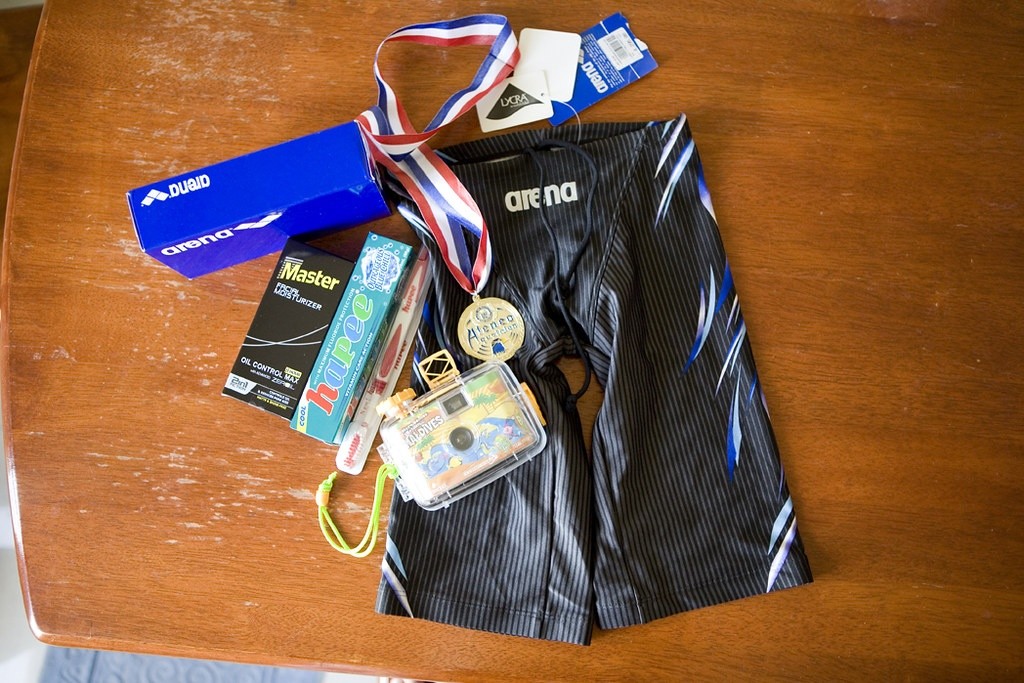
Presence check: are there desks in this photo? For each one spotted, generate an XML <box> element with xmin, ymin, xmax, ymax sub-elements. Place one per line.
<box><xmin>0</xmin><ymin>0</ymin><xmax>1024</xmax><ymax>683</ymax></box>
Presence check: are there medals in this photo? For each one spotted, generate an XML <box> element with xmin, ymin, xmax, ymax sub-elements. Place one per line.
<box><xmin>458</xmin><ymin>297</ymin><xmax>525</xmax><ymax>362</ymax></box>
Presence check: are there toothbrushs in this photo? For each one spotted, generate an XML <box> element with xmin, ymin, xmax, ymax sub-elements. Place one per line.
<box><xmin>334</xmin><ymin>245</ymin><xmax>430</xmax><ymax>476</ymax></box>
